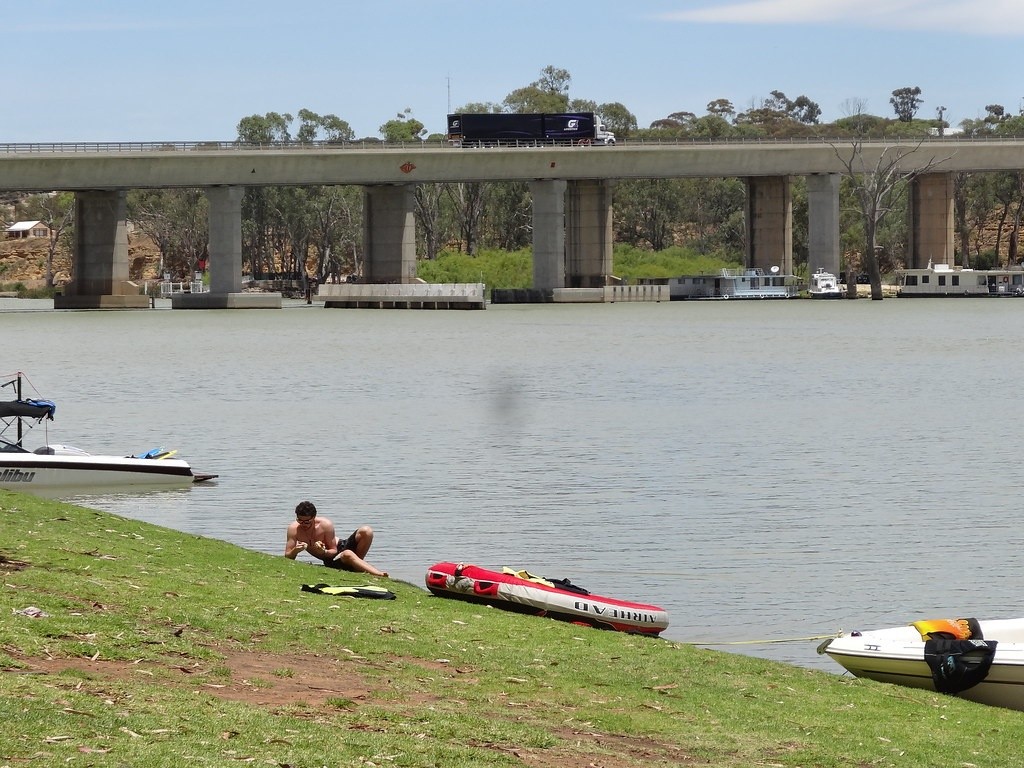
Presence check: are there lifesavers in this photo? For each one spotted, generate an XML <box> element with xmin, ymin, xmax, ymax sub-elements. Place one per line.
<box><xmin>785</xmin><ymin>293</ymin><xmax>791</xmax><ymax>299</ymax></box>
<box><xmin>759</xmin><ymin>294</ymin><xmax>766</xmax><ymax>299</ymax></box>
<box><xmin>724</xmin><ymin>295</ymin><xmax>729</xmax><ymax>299</ymax></box>
<box><xmin>1003</xmin><ymin>276</ymin><xmax>1010</xmax><ymax>283</ymax></box>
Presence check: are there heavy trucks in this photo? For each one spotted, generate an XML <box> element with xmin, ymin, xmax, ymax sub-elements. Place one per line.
<box><xmin>445</xmin><ymin>109</ymin><xmax>616</xmax><ymax>147</ymax></box>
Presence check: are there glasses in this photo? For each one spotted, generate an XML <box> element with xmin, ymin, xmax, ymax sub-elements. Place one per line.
<box><xmin>296</xmin><ymin>516</ymin><xmax>314</xmax><ymax>524</ymax></box>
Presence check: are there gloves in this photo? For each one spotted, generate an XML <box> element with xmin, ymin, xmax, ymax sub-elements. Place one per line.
<box><xmin>315</xmin><ymin>541</ymin><xmax>327</xmax><ymax>555</ymax></box>
<box><xmin>293</xmin><ymin>540</ymin><xmax>308</xmax><ymax>553</ymax></box>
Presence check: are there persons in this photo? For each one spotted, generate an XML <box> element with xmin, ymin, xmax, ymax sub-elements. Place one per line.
<box><xmin>285</xmin><ymin>501</ymin><xmax>388</xmax><ymax>577</ymax></box>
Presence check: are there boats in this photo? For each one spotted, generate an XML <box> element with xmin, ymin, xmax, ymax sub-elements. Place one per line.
<box><xmin>684</xmin><ymin>273</ymin><xmax>802</xmax><ymax>300</ymax></box>
<box><xmin>0</xmin><ymin>370</ymin><xmax>215</xmax><ymax>491</ymax></box>
<box><xmin>894</xmin><ymin>256</ymin><xmax>1024</xmax><ymax>298</ymax></box>
<box><xmin>807</xmin><ymin>267</ymin><xmax>844</xmax><ymax>299</ymax></box>
<box><xmin>818</xmin><ymin>616</ymin><xmax>1024</xmax><ymax>711</ymax></box>
<box><xmin>424</xmin><ymin>560</ymin><xmax>670</xmax><ymax>641</ymax></box>
